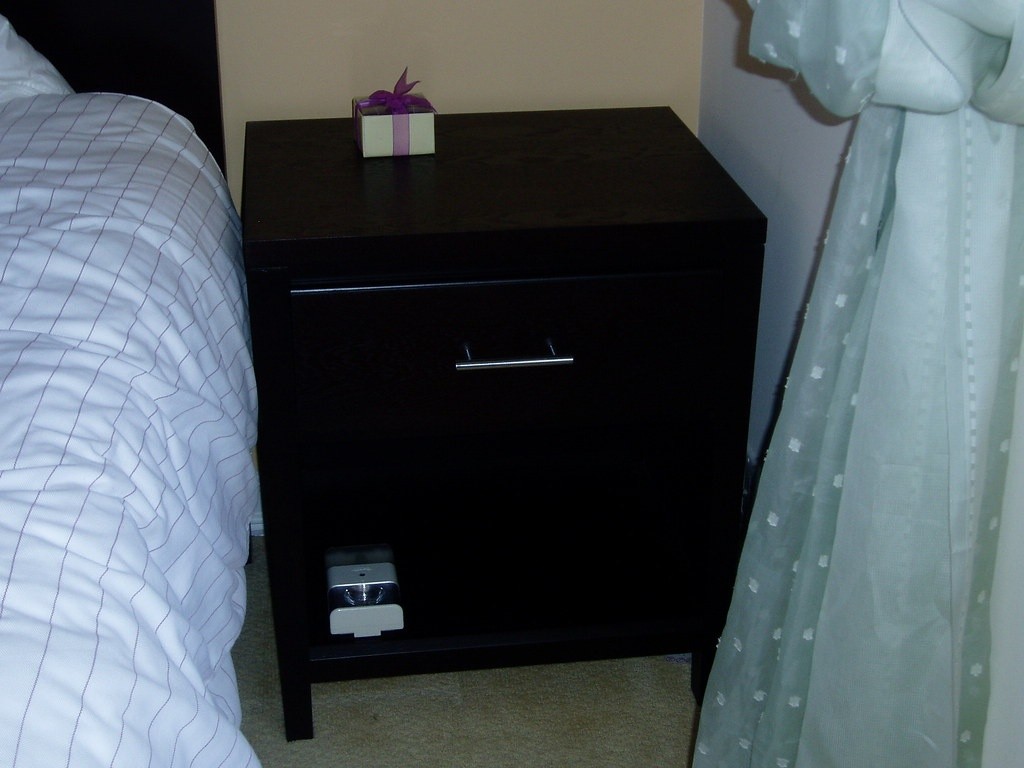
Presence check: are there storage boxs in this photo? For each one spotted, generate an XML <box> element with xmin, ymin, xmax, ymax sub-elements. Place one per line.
<box><xmin>352</xmin><ymin>92</ymin><xmax>436</xmax><ymax>158</ymax></box>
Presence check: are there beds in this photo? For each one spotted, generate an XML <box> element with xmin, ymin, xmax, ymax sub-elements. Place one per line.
<box><xmin>0</xmin><ymin>0</ymin><xmax>263</xmax><ymax>768</ymax></box>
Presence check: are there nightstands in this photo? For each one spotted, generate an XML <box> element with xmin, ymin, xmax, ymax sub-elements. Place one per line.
<box><xmin>239</xmin><ymin>106</ymin><xmax>768</xmax><ymax>742</ymax></box>
